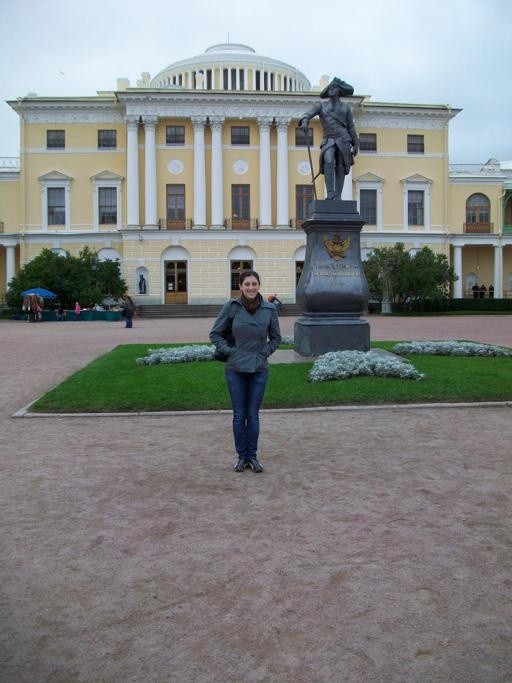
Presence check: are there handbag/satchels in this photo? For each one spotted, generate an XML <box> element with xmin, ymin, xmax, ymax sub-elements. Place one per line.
<box><xmin>214</xmin><ymin>318</ymin><xmax>235</xmax><ymax>361</ymax></box>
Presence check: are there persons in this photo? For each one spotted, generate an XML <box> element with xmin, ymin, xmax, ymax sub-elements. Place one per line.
<box><xmin>472</xmin><ymin>282</ymin><xmax>494</xmax><ymax>298</ymax></box>
<box><xmin>208</xmin><ymin>268</ymin><xmax>281</xmax><ymax>472</ymax></box>
<box><xmin>74</xmin><ymin>302</ymin><xmax>81</xmax><ymax>320</ymax></box>
<box><xmin>121</xmin><ymin>293</ymin><xmax>136</xmax><ymax>328</ymax></box>
<box><xmin>271</xmin><ymin>294</ymin><xmax>281</xmax><ymax>305</ymax></box>
<box><xmin>297</xmin><ymin>76</ymin><xmax>360</xmax><ymax>201</ymax></box>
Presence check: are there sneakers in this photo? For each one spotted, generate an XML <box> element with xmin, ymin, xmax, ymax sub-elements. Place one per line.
<box><xmin>234</xmin><ymin>459</ymin><xmax>246</xmax><ymax>471</ymax></box>
<box><xmin>247</xmin><ymin>459</ymin><xmax>263</xmax><ymax>472</ymax></box>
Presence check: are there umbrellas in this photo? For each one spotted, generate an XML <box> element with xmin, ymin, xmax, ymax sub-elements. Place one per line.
<box><xmin>20</xmin><ymin>286</ymin><xmax>57</xmax><ymax>320</ymax></box>
